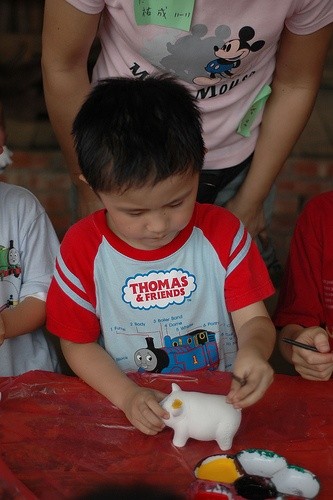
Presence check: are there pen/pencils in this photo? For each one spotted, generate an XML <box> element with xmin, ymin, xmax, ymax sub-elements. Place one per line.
<box><xmin>278</xmin><ymin>336</ymin><xmax>332</xmax><ymax>354</ymax></box>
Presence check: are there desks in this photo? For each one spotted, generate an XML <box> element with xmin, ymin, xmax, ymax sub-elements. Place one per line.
<box><xmin>0</xmin><ymin>370</ymin><xmax>333</xmax><ymax>500</ymax></box>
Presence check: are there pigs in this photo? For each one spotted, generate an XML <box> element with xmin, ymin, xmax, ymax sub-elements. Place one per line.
<box><xmin>157</xmin><ymin>383</ymin><xmax>242</xmax><ymax>450</ymax></box>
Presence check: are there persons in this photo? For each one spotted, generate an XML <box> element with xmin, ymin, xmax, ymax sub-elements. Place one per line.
<box><xmin>41</xmin><ymin>0</ymin><xmax>333</xmax><ymax>292</ymax></box>
<box><xmin>45</xmin><ymin>72</ymin><xmax>277</xmax><ymax>436</ymax></box>
<box><xmin>272</xmin><ymin>189</ymin><xmax>333</xmax><ymax>381</ymax></box>
<box><xmin>0</xmin><ymin>126</ymin><xmax>74</xmax><ymax>377</ymax></box>
